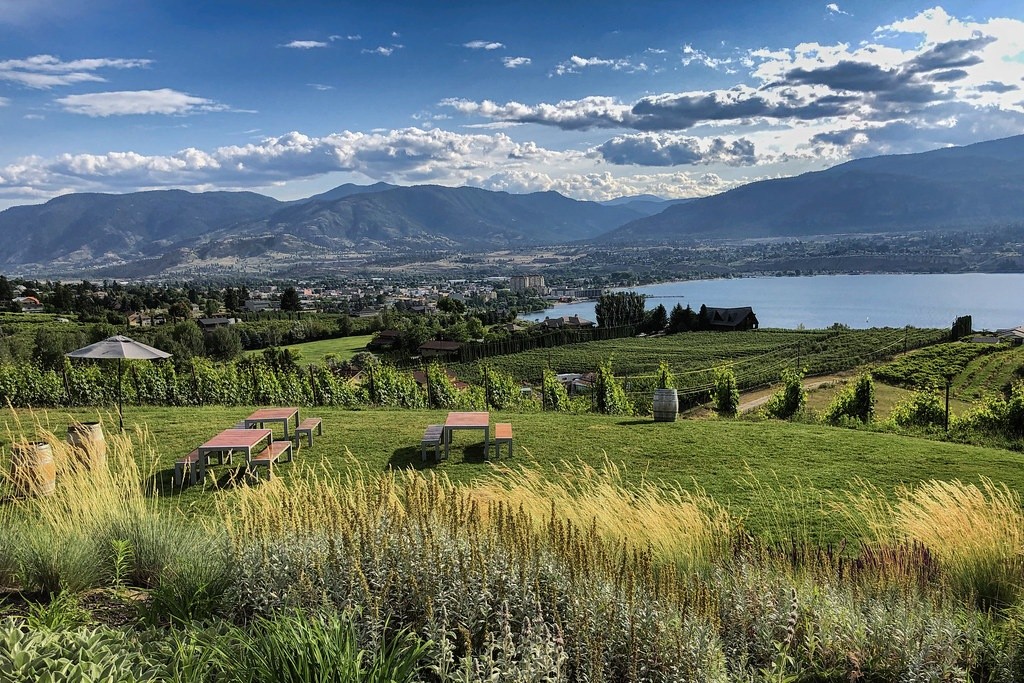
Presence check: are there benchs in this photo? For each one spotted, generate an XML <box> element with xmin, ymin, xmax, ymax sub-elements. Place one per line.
<box><xmin>423</xmin><ymin>424</ymin><xmax>444</xmax><ymax>461</ymax></box>
<box><xmin>233</xmin><ymin>422</ymin><xmax>254</xmax><ymax>429</ymax></box>
<box><xmin>251</xmin><ymin>441</ymin><xmax>293</xmax><ymax>480</ymax></box>
<box><xmin>296</xmin><ymin>418</ymin><xmax>323</xmax><ymax>447</ymax></box>
<box><xmin>174</xmin><ymin>446</ymin><xmax>212</xmax><ymax>484</ymax></box>
<box><xmin>495</xmin><ymin>423</ymin><xmax>512</xmax><ymax>458</ymax></box>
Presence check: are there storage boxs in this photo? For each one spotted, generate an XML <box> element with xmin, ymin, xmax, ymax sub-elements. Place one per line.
<box><xmin>68</xmin><ymin>422</ymin><xmax>104</xmax><ymax>470</ymax></box>
<box><xmin>654</xmin><ymin>389</ymin><xmax>678</xmax><ymax>421</ymax></box>
<box><xmin>11</xmin><ymin>443</ymin><xmax>56</xmax><ymax>495</ymax></box>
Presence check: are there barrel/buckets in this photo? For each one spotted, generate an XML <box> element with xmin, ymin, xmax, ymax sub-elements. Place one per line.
<box><xmin>10</xmin><ymin>441</ymin><xmax>57</xmax><ymax>498</ymax></box>
<box><xmin>653</xmin><ymin>389</ymin><xmax>678</xmax><ymax>422</ymax></box>
<box><xmin>67</xmin><ymin>421</ymin><xmax>106</xmax><ymax>471</ymax></box>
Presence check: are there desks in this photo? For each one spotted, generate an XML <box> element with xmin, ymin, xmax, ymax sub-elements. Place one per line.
<box><xmin>246</xmin><ymin>408</ymin><xmax>299</xmax><ymax>449</ymax></box>
<box><xmin>444</xmin><ymin>412</ymin><xmax>489</xmax><ymax>460</ymax></box>
<box><xmin>199</xmin><ymin>429</ymin><xmax>273</xmax><ymax>485</ymax></box>
